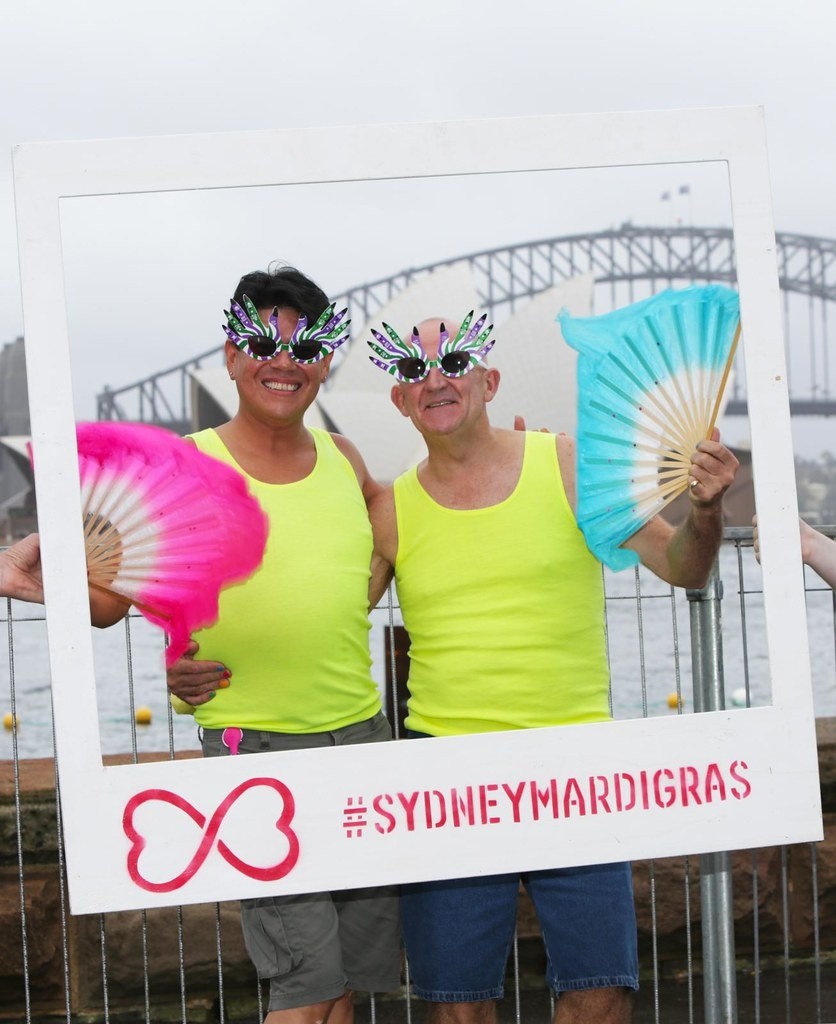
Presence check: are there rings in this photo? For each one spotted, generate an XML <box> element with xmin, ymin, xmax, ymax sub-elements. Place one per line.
<box><xmin>690</xmin><ymin>479</ymin><xmax>700</xmax><ymax>487</ymax></box>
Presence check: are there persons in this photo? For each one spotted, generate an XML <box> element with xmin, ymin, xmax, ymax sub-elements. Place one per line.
<box><xmin>89</xmin><ymin>260</ymin><xmax>572</xmax><ymax>1024</ymax></box>
<box><xmin>752</xmin><ymin>507</ymin><xmax>836</xmax><ymax>591</ymax></box>
<box><xmin>1</xmin><ymin>533</ymin><xmax>45</xmax><ymax>606</ymax></box>
<box><xmin>170</xmin><ymin>308</ymin><xmax>740</xmax><ymax>1024</ymax></box>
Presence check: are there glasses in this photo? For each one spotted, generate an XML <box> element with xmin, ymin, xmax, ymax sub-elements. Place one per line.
<box><xmin>222</xmin><ymin>294</ymin><xmax>352</xmax><ymax>365</ymax></box>
<box><xmin>367</xmin><ymin>310</ymin><xmax>496</xmax><ymax>383</ymax></box>
